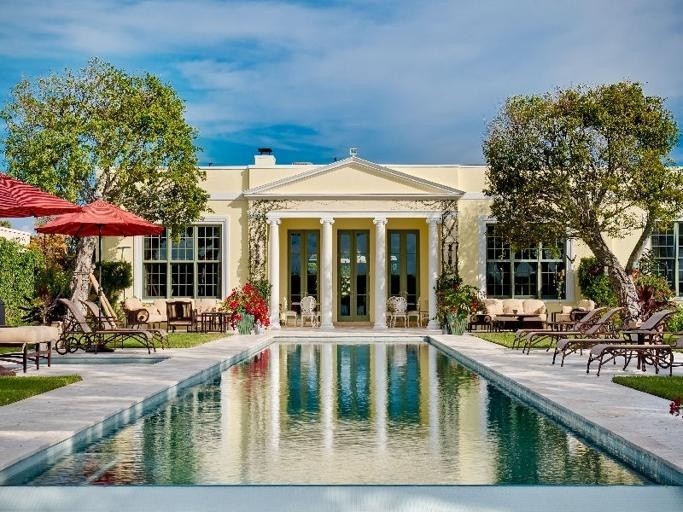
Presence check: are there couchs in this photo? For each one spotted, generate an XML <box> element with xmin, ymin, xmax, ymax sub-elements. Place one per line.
<box><xmin>481</xmin><ymin>299</ymin><xmax>548</xmax><ymax>329</ymax></box>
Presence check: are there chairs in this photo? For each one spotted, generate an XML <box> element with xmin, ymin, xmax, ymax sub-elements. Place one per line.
<box><xmin>512</xmin><ymin>307</ymin><xmax>678</xmax><ymax>376</ymax></box>
<box><xmin>388</xmin><ymin>296</ymin><xmax>420</xmax><ymax>328</ymax></box>
<box><xmin>120</xmin><ymin>296</ymin><xmax>198</xmax><ymax>333</ymax></box>
<box><xmin>282</xmin><ymin>295</ymin><xmax>318</xmax><ymax>328</ymax></box>
<box><xmin>55</xmin><ymin>298</ymin><xmax>169</xmax><ymax>355</ymax></box>
<box><xmin>0</xmin><ymin>325</ymin><xmax>59</xmax><ymax>372</ymax></box>
<box><xmin>550</xmin><ymin>298</ymin><xmax>595</xmax><ymax>332</ymax></box>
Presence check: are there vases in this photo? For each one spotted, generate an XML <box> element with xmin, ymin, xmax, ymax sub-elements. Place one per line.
<box><xmin>445</xmin><ymin>313</ymin><xmax>468</xmax><ymax>335</ymax></box>
<box><xmin>235</xmin><ymin>314</ymin><xmax>255</xmax><ymax>335</ymax></box>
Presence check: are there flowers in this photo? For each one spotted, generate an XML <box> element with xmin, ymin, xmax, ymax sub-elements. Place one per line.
<box><xmin>429</xmin><ymin>285</ymin><xmax>491</xmax><ymax>328</ymax></box>
<box><xmin>218</xmin><ymin>283</ymin><xmax>270</xmax><ymax>330</ymax></box>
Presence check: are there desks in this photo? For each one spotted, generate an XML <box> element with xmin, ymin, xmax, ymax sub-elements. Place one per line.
<box><xmin>202</xmin><ymin>312</ymin><xmax>232</xmax><ymax>333</ymax></box>
<box><xmin>496</xmin><ymin>315</ymin><xmax>540</xmax><ymax>329</ymax></box>
<box><xmin>420</xmin><ymin>311</ymin><xmax>429</xmax><ymax>327</ymax></box>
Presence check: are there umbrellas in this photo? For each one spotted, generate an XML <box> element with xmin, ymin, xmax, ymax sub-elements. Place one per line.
<box><xmin>35</xmin><ymin>197</ymin><xmax>165</xmax><ymax>332</ymax></box>
<box><xmin>0</xmin><ymin>171</ymin><xmax>81</xmax><ymax>218</ymax></box>
